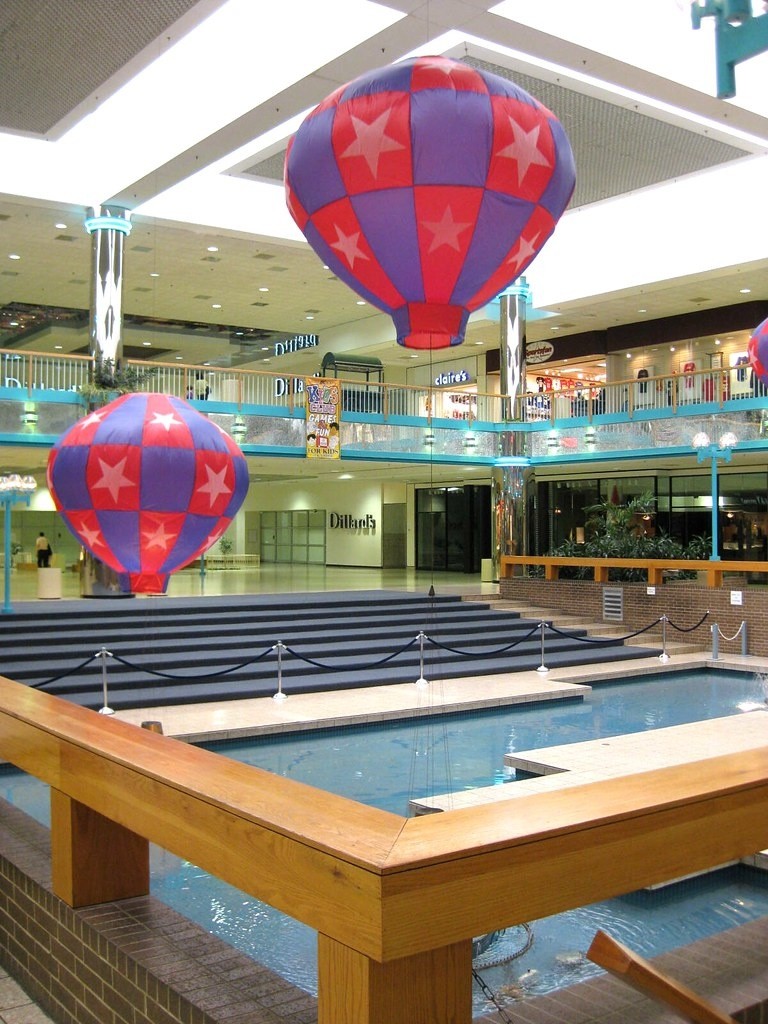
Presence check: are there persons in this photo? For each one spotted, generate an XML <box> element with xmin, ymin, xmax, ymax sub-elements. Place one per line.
<box><xmin>35</xmin><ymin>532</ymin><xmax>48</xmax><ymax>568</ymax></box>
<box><xmin>194</xmin><ymin>372</ymin><xmax>209</xmax><ymax>400</ymax></box>
<box><xmin>186</xmin><ymin>385</ymin><xmax>193</xmax><ymax>399</ymax></box>
